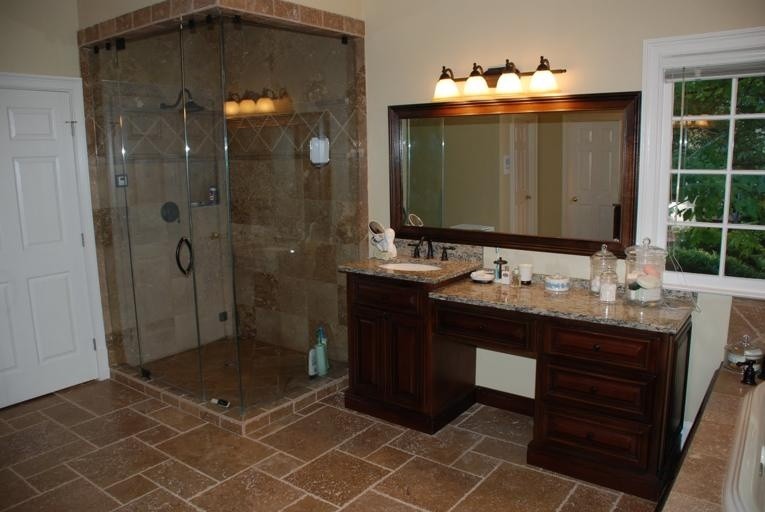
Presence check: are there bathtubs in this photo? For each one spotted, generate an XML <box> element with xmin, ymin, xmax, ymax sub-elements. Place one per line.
<box><xmin>724</xmin><ymin>382</ymin><xmax>765</xmax><ymax>512</ymax></box>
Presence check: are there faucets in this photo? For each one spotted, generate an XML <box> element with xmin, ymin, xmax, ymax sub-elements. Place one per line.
<box><xmin>420</xmin><ymin>235</ymin><xmax>435</xmax><ymax>258</ymax></box>
<box><xmin>736</xmin><ymin>360</ymin><xmax>764</xmax><ymax>383</ymax></box>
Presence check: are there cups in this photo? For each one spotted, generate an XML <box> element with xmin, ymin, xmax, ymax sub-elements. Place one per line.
<box><xmin>519</xmin><ymin>263</ymin><xmax>534</xmax><ymax>285</ymax></box>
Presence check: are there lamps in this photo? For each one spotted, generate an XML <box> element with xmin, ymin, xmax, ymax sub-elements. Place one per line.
<box><xmin>432</xmin><ymin>56</ymin><xmax>567</xmax><ymax>101</ymax></box>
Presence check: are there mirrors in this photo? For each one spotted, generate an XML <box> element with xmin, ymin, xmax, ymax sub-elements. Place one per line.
<box><xmin>388</xmin><ymin>91</ymin><xmax>642</xmax><ymax>261</ymax></box>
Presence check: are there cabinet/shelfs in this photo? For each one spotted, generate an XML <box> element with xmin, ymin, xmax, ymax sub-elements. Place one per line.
<box><xmin>431</xmin><ymin>298</ymin><xmax>539</xmax><ymax>359</ymax></box>
<box><xmin>344</xmin><ymin>272</ymin><xmax>475</xmax><ymax>436</ymax></box>
<box><xmin>527</xmin><ymin>314</ymin><xmax>693</xmax><ymax>504</ymax></box>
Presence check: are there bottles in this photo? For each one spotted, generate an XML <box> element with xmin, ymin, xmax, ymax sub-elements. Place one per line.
<box><xmin>590</xmin><ymin>243</ymin><xmax>618</xmax><ymax>299</ymax></box>
<box><xmin>599</xmin><ymin>265</ymin><xmax>618</xmax><ymax>305</ymax></box>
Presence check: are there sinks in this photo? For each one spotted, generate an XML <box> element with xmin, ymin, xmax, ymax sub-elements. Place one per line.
<box><xmin>379</xmin><ymin>262</ymin><xmax>442</xmax><ymax>272</ymax></box>
